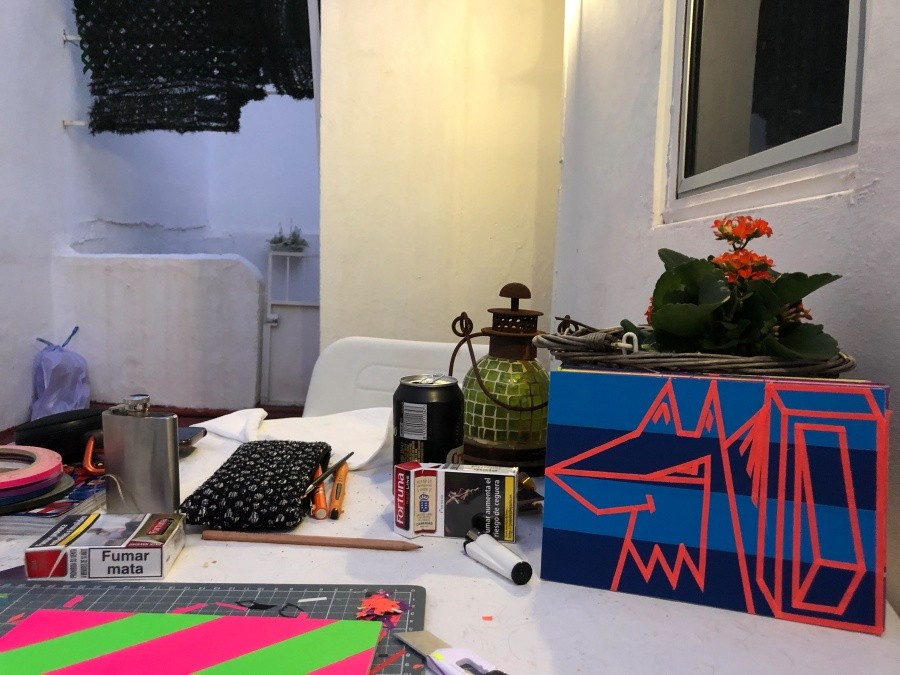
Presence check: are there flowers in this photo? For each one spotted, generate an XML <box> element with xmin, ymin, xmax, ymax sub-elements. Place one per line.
<box><xmin>645</xmin><ymin>216</ymin><xmax>846</xmax><ymax>365</ymax></box>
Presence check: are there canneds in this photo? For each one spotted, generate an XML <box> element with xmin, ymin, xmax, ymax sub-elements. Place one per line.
<box><xmin>393</xmin><ymin>374</ymin><xmax>464</xmax><ymax>466</ymax></box>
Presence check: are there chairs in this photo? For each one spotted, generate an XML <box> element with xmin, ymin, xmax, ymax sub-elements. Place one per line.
<box><xmin>297</xmin><ymin>334</ymin><xmax>491</xmax><ymax>418</ymax></box>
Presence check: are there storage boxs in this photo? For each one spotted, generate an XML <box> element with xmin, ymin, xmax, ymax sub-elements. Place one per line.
<box><xmin>537</xmin><ymin>367</ymin><xmax>888</xmax><ymax>640</ymax></box>
<box><xmin>393</xmin><ymin>457</ymin><xmax>521</xmax><ymax>544</ymax></box>
<box><xmin>23</xmin><ymin>514</ymin><xmax>186</xmax><ymax>579</ymax></box>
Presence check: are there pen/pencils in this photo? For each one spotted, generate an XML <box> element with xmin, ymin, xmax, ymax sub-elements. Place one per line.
<box><xmin>200</xmin><ymin>529</ymin><xmax>425</xmax><ymax>551</ymax></box>
<box><xmin>305</xmin><ymin>451</ymin><xmax>355</xmax><ymax>496</ymax></box>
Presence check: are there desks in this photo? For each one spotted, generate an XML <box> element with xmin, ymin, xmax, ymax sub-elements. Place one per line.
<box><xmin>0</xmin><ymin>412</ymin><xmax>898</xmax><ymax>674</ymax></box>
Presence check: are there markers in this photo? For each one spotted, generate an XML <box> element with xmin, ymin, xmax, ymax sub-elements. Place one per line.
<box><xmin>329</xmin><ymin>462</ymin><xmax>348</xmax><ymax>520</ymax></box>
<box><xmin>310</xmin><ymin>464</ymin><xmax>327</xmax><ymax>519</ymax></box>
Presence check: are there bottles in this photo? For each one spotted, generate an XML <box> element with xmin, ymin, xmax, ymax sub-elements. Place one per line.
<box><xmin>101</xmin><ymin>393</ymin><xmax>180</xmax><ymax>519</ymax></box>
